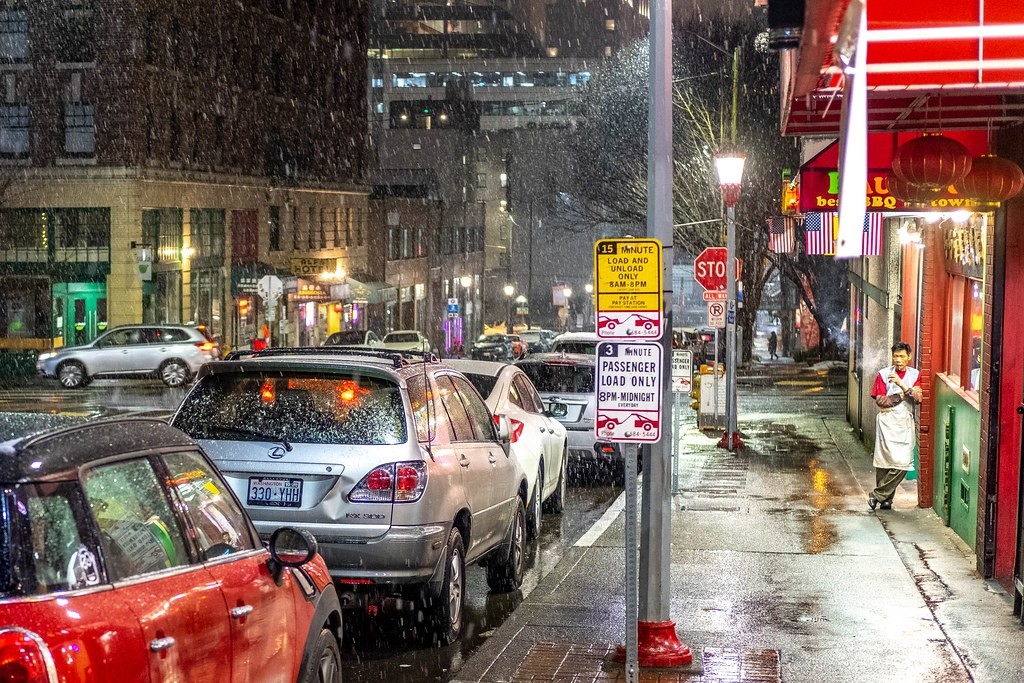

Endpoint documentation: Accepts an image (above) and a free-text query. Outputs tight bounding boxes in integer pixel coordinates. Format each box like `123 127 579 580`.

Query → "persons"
767 331 779 361
867 341 923 511
253 313 271 352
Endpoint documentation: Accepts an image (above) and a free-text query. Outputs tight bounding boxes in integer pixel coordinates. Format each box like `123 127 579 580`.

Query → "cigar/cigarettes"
898 365 900 370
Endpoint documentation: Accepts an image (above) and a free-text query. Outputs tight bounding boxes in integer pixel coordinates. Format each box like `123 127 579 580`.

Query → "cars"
434 356 570 539
700 329 726 362
549 331 644 355
472 334 514 362
1 410 344 683
382 330 431 352
36 323 223 390
320 329 386 348
673 329 708 372
505 335 529 359
168 346 529 645
518 330 556 354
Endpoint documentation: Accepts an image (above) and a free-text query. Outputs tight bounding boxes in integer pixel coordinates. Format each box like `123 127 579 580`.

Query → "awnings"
346 272 397 304
230 259 298 296
290 273 350 302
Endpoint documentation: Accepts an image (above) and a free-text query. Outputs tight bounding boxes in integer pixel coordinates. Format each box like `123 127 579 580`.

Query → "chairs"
275 391 329 429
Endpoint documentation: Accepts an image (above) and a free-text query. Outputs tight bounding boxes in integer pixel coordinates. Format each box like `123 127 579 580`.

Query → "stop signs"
694 247 740 291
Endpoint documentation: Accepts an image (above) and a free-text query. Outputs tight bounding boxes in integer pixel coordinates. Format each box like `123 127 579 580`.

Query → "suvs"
511 349 642 486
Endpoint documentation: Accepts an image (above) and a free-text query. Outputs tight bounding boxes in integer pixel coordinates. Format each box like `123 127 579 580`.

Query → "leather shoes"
869 491 877 510
880 500 891 509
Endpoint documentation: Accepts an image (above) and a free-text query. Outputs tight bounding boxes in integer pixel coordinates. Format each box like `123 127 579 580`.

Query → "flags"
804 210 883 256
766 215 795 254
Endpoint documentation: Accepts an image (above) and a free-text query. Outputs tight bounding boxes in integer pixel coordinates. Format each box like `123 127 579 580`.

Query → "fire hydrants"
689 365 714 428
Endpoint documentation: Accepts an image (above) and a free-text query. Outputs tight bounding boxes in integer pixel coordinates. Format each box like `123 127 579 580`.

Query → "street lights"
715 152 747 450
504 286 514 336
586 284 593 332
564 289 572 332
459 274 472 346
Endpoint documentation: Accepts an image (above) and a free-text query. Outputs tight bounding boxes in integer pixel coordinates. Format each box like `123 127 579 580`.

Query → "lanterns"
892 132 972 188
953 154 1024 210
886 167 948 208
